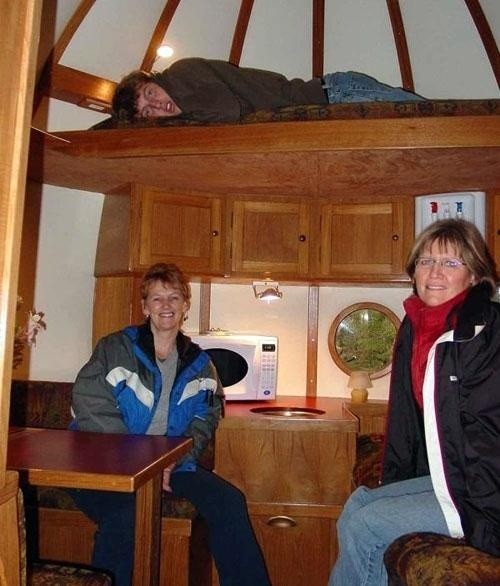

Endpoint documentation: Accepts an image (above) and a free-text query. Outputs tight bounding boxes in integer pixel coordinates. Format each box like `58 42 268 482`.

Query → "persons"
325 220 500 586
67 263 272 586
112 58 427 123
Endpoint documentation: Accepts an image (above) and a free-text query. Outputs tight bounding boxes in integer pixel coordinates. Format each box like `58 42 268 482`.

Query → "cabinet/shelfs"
93 182 500 287
210 429 356 586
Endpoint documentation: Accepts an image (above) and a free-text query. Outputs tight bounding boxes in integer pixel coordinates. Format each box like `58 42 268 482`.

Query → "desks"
6 423 194 586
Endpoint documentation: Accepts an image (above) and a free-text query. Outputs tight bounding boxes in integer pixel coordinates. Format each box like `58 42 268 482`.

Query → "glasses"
414 257 467 270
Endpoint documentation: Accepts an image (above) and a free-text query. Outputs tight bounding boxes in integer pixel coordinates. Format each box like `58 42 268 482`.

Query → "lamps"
253 279 283 304
140 44 173 76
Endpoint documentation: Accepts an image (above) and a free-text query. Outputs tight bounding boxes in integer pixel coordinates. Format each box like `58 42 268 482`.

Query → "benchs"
38 507 191 586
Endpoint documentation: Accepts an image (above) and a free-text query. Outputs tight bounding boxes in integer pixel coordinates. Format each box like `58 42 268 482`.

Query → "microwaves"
182 336 279 401
